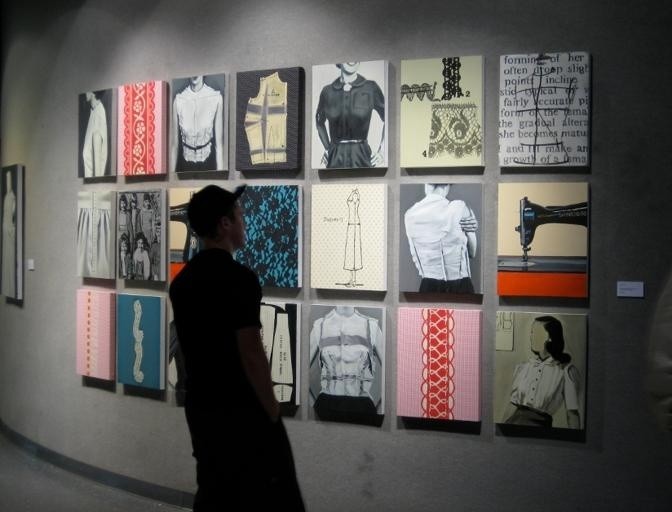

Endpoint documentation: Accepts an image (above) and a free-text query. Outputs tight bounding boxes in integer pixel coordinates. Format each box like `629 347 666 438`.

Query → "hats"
187 185 246 235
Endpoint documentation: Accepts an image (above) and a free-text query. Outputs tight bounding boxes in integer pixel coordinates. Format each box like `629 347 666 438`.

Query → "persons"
308 306 384 414
316 61 385 169
168 183 305 512
501 315 581 431
404 183 478 295
118 193 161 283
82 89 108 178
1 169 16 300
169 75 223 170
